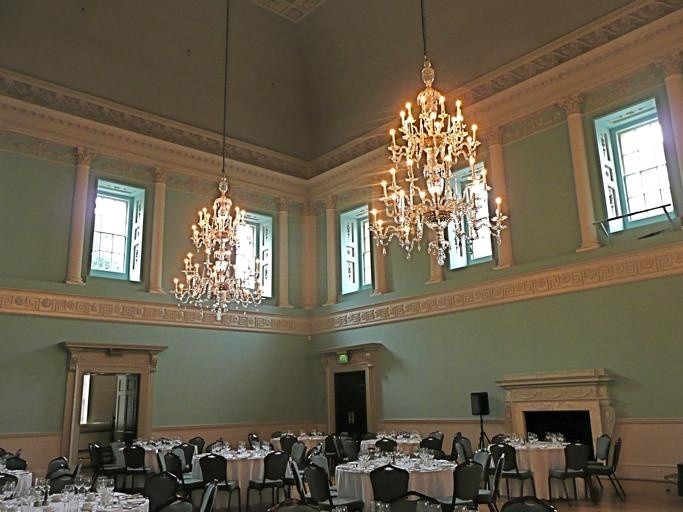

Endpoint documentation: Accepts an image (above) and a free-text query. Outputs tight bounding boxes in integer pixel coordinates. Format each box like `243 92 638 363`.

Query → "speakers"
471 390 492 417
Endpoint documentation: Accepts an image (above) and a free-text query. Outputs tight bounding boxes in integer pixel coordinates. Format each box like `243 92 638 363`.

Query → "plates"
441 464 454 467
423 468 438 470
435 460 446 462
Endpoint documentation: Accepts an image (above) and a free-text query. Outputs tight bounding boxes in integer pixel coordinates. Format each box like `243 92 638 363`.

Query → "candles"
187 252 193 265
405 101 411 116
400 110 405 125
372 208 376 222
381 180 387 196
390 167 396 184
456 98 461 115
195 264 198 272
471 122 477 141
184 259 188 269
439 96 445 112
496 196 502 209
389 128 395 145
198 210 203 221
174 277 179 289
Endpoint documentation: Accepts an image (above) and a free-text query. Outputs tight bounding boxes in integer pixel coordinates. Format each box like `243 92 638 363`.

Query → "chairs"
272 431 283 438
429 449 445 459
207 440 223 452
389 491 439 512
291 461 338 504
0 447 28 470
436 459 483 512
305 463 364 512
285 440 308 497
587 433 611 488
45 456 83 496
375 436 397 452
420 436 442 450
88 440 125 490
189 436 205 454
143 471 179 512
474 448 491 490
429 431 444 441
171 446 193 479
458 436 474 460
370 462 409 504
248 434 262 450
199 453 241 512
343 439 358 461
246 450 289 510
325 433 339 473
478 453 506 512
199 478 218 512
123 444 155 489
153 494 193 512
306 447 318 463
548 440 598 507
446 432 462 461
490 442 536 497
310 456 338 491
500 495 556 512
333 438 349 464
164 452 204 499
456 441 466 463
177 442 195 472
0 472 18 500
267 498 320 512
156 449 165 473
585 437 626 501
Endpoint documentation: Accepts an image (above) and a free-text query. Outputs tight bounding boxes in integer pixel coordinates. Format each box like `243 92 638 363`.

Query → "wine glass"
557 433 561 445
255 442 260 451
430 504 442 512
550 434 557 445
252 441 256 448
366 455 370 461
30 491 43 512
362 449 370 459
60 489 68 512
422 448 429 456
4 481 15 500
413 446 420 459
503 432 507 444
454 505 467 512
74 477 84 494
311 428 316 436
546 432 551 440
530 436 534 448
404 446 410 455
358 451 364 461
365 500 392 512
224 440 232 451
98 476 107 489
512 432 516 440
301 428 306 436
532 434 538 443
428 449 435 459
103 478 115 508
515 433 519 442
262 440 268 449
528 432 532 440
416 500 428 512
336 506 347 512
65 485 75 512
238 440 245 448
507 433 511 441
35 477 43 487
83 475 92 492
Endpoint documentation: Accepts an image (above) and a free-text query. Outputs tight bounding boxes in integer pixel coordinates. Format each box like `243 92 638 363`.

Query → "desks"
117 444 199 489
488 442 590 500
270 436 328 455
0 470 32 495
0 493 149 512
192 452 293 511
361 439 421 454
334 458 458 511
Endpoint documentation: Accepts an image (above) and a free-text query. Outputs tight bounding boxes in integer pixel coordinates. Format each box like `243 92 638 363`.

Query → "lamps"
169 1 263 320
370 0 509 266
